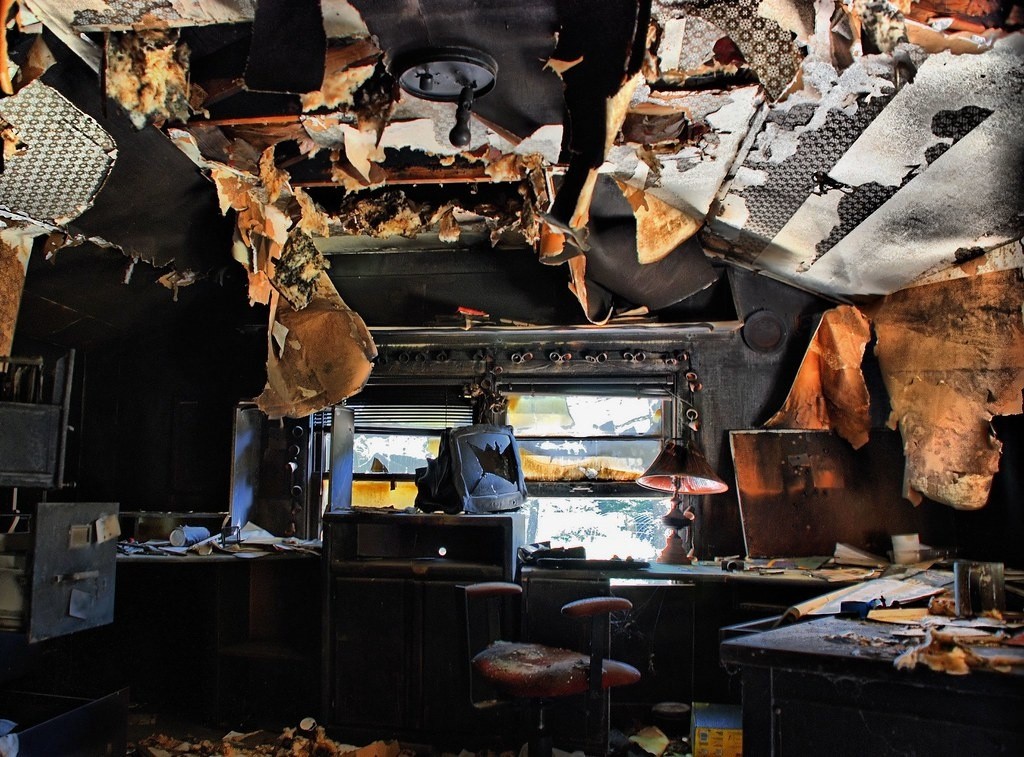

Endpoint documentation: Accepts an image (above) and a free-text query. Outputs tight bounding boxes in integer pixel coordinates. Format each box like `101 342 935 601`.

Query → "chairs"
454 581 641 757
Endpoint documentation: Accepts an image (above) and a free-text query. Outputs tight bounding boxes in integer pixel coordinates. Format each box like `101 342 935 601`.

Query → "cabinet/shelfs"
719 580 1024 757
519 564 731 757
319 576 520 754
